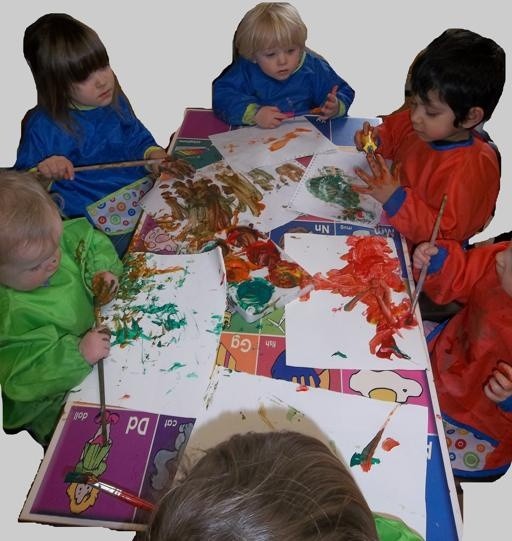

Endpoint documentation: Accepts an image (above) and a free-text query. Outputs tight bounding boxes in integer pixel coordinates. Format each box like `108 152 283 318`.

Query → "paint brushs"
63 472 155 513
405 194 447 324
93 296 106 444
74 157 178 172
276 108 321 120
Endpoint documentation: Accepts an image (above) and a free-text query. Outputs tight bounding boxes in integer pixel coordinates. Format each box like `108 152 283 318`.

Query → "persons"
210 1 355 130
349 27 505 259
0 165 125 447
145 431 377 541
409 238 511 483
13 13 195 258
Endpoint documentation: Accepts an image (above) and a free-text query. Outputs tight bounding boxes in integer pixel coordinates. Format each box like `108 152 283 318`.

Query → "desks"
15 106 467 539
